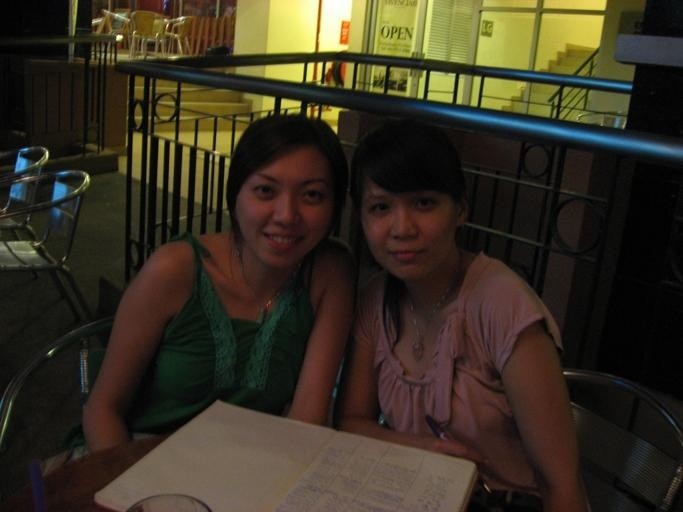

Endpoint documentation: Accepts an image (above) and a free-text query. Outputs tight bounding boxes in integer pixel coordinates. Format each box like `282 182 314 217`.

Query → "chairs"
0 146 49 281
95 8 193 59
378 369 683 511
0 316 111 452
0 169 92 322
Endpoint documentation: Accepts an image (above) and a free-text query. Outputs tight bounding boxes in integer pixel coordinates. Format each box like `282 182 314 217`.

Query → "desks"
1 398 164 512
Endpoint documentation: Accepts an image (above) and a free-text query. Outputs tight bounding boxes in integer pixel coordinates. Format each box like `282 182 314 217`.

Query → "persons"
62 113 361 452
332 113 588 512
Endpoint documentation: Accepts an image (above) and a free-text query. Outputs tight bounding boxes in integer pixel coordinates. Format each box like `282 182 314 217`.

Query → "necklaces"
235 245 304 325
404 248 464 358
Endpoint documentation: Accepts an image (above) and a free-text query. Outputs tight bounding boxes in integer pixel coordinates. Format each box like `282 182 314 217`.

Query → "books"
93 398 480 512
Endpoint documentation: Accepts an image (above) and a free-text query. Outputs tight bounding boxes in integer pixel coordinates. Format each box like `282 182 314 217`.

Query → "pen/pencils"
424 414 494 495
29 459 44 512
